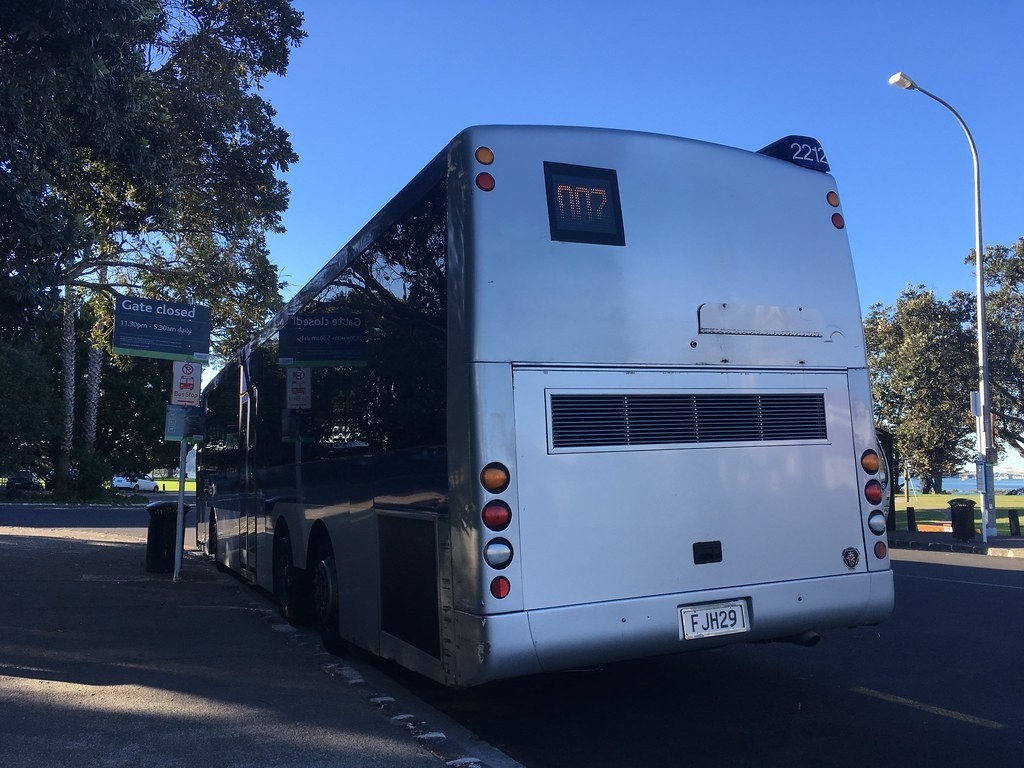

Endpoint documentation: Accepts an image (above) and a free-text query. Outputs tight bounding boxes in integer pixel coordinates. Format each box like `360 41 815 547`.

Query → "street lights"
888 69 997 546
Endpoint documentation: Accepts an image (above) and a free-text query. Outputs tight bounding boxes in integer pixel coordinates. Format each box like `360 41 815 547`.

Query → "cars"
6 469 42 489
45 468 80 491
111 472 159 493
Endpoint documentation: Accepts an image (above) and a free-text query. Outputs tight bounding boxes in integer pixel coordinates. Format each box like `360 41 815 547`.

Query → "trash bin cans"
946 498 978 542
142 499 193 576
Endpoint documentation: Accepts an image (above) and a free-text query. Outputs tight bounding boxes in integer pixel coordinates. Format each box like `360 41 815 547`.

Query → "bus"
193 121 899 696
